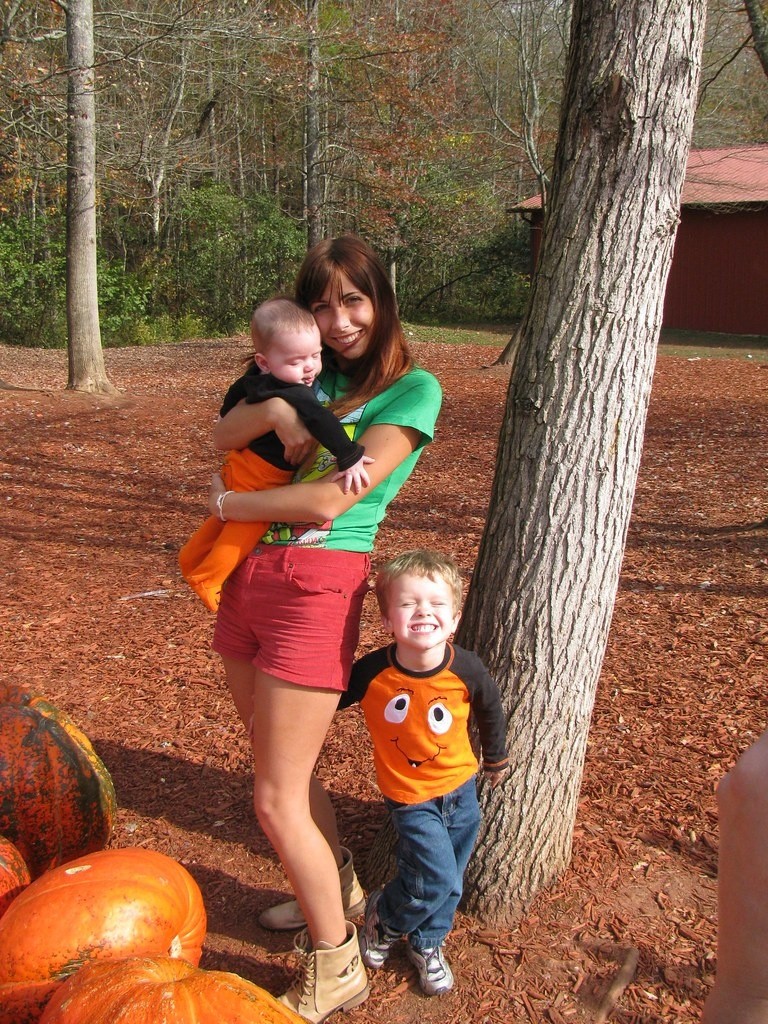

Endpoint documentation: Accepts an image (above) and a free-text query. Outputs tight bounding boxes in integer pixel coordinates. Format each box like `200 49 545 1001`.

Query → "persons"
179 298 376 611
336 549 510 995
209 235 442 1024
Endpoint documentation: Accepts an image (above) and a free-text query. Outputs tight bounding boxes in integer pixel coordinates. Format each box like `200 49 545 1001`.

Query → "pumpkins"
0 679 319 1024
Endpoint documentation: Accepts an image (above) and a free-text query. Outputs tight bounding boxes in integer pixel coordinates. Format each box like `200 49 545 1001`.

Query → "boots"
276 920 370 1024
259 845 366 933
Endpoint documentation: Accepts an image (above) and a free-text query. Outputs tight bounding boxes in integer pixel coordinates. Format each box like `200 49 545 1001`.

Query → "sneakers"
358 889 403 969
406 941 455 996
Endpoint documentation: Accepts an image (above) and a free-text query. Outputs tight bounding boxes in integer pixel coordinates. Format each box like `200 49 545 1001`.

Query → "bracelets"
216 491 235 521
